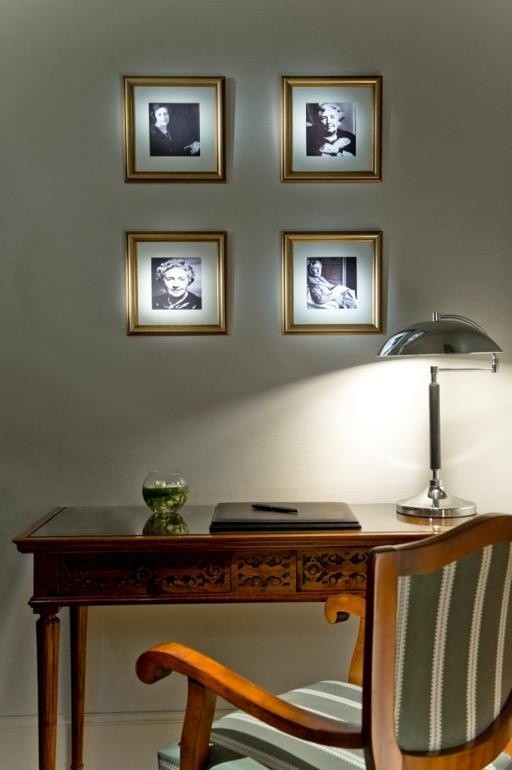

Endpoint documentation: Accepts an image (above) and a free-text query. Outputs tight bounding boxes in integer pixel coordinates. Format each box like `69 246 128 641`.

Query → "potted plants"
142 470 188 512
143 512 188 535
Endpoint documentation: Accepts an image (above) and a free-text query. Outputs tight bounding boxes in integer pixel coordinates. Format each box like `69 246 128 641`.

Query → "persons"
307 103 356 157
152 257 202 310
149 103 200 156
308 260 357 310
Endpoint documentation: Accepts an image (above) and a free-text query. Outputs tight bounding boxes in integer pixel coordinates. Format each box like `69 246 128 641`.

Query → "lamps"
376 313 503 518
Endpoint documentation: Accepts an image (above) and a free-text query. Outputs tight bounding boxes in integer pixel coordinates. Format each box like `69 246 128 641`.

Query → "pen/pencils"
252 504 298 512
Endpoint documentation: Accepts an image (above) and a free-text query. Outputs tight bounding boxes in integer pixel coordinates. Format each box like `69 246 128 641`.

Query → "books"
209 501 362 531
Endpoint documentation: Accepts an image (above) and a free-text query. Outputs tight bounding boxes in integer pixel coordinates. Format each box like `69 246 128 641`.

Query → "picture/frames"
281 75 383 184
281 231 383 334
125 232 227 336
123 77 227 184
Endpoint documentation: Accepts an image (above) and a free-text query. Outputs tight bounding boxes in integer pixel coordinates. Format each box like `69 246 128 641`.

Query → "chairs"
135 512 512 770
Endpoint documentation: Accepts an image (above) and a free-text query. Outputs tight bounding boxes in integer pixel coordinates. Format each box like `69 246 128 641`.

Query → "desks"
12 504 480 770
